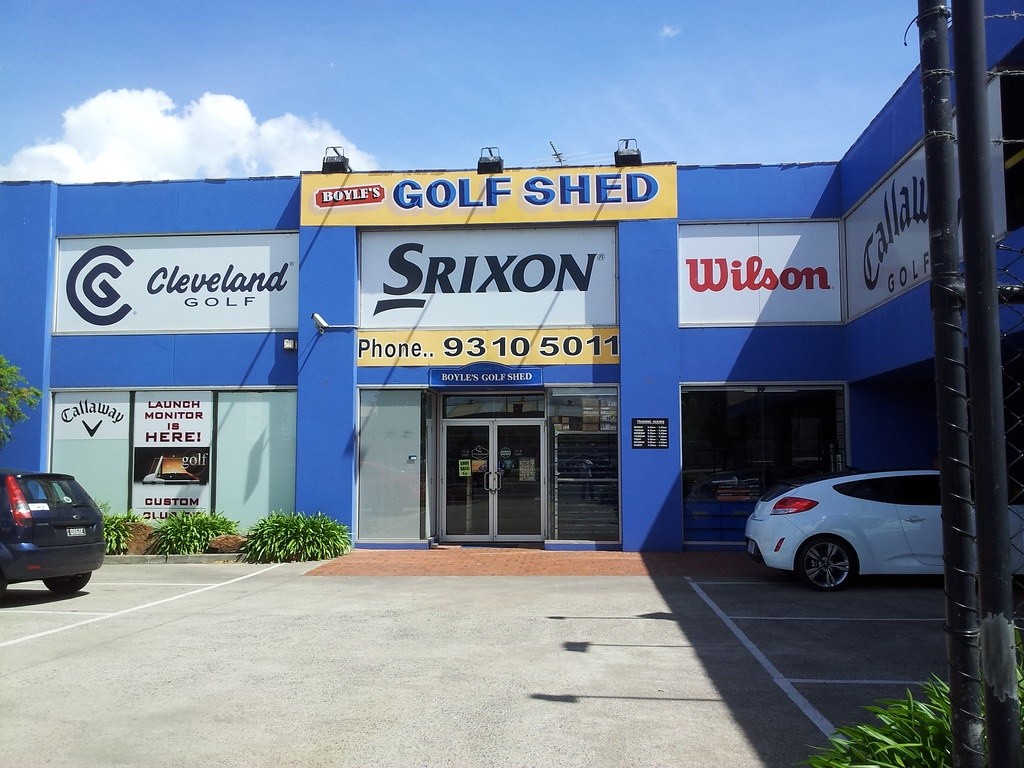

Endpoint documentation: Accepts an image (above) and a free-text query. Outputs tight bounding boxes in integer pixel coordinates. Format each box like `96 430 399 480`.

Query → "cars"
746 471 1024 593
0 468 107 595
685 467 777 502
